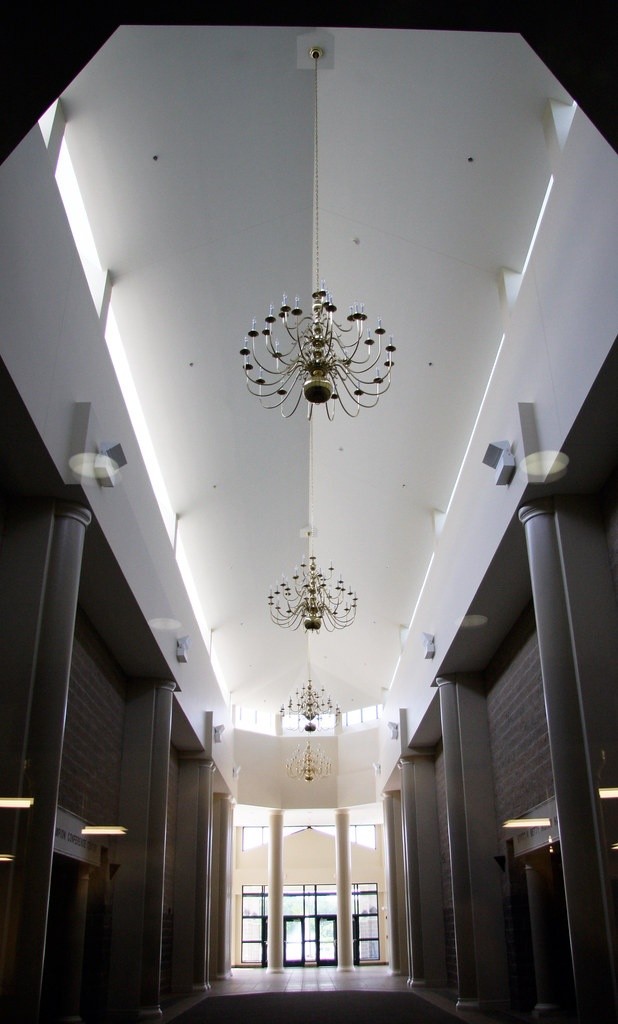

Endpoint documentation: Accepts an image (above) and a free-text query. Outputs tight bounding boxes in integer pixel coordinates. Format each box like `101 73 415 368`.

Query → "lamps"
265 530 357 636
235 31 399 423
285 742 330 783
278 676 344 734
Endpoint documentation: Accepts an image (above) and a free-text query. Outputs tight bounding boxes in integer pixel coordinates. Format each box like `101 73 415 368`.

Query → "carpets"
171 990 472 1024
